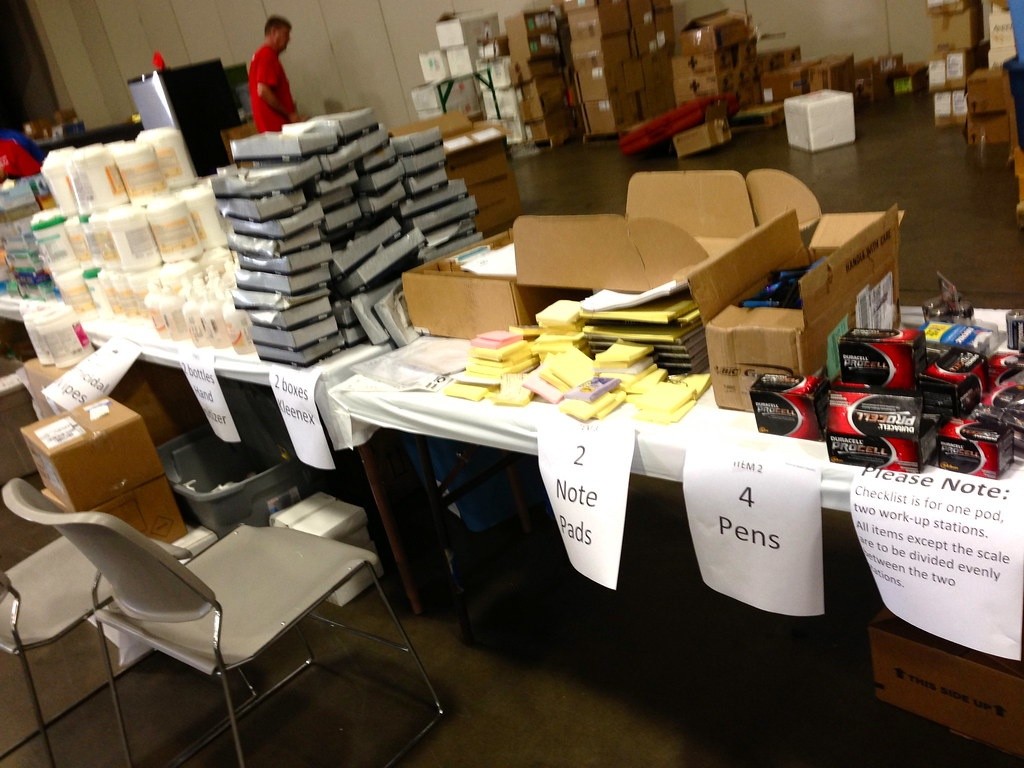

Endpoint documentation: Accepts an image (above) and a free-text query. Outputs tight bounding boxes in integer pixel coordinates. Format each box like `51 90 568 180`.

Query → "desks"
1 293 423 617
339 325 1024 643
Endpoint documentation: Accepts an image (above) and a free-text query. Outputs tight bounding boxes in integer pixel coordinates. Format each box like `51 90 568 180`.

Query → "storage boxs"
0 0 1024 768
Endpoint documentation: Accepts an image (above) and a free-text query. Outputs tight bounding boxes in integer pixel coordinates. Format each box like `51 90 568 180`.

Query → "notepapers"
442 299 713 426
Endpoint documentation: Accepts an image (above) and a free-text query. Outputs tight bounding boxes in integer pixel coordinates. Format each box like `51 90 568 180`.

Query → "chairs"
0 533 256 768
0 477 446 767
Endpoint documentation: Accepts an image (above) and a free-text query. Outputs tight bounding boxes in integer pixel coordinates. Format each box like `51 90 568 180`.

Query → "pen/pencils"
739 299 781 310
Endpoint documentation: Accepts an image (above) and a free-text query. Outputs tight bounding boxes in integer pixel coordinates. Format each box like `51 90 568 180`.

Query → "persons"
247 15 307 134
0 129 45 183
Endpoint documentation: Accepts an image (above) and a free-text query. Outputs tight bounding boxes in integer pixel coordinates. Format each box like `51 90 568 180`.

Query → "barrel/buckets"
22 130 258 369
